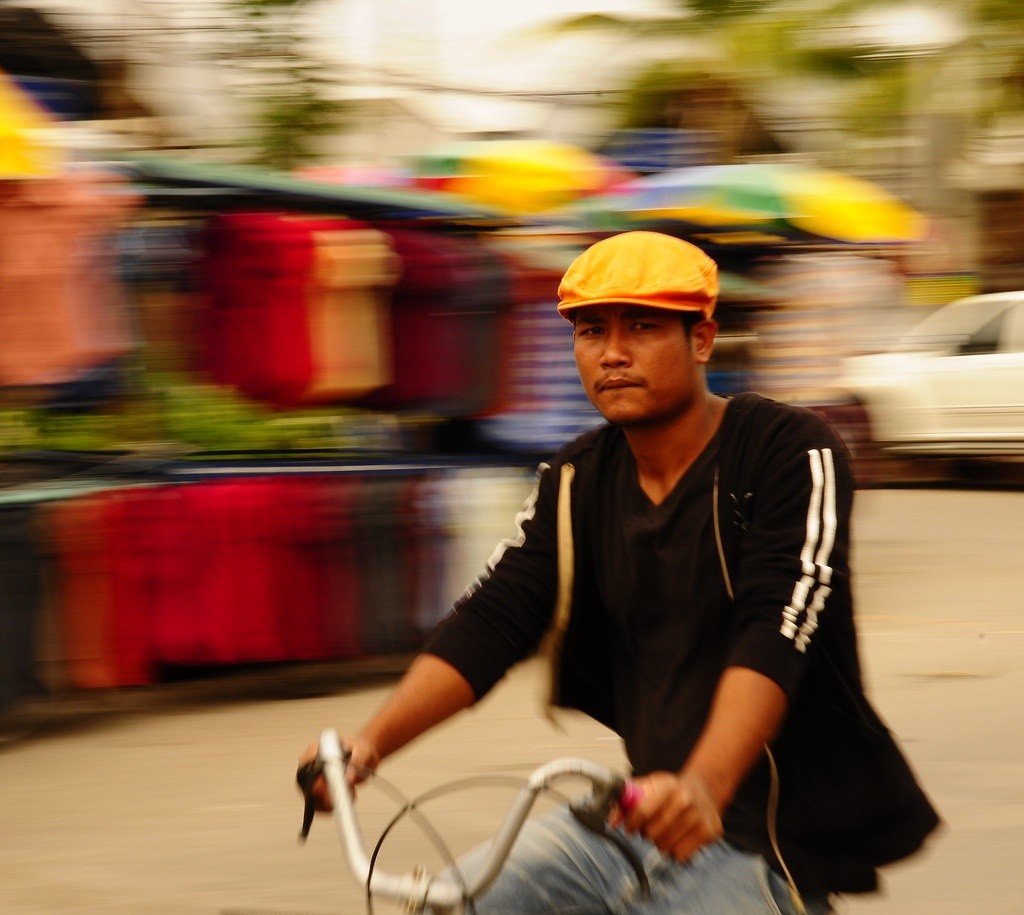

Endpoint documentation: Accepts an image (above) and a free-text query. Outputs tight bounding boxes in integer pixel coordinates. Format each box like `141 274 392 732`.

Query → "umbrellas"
387 136 934 248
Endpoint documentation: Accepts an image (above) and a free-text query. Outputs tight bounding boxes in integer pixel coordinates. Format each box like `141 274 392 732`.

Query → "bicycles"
296 730 695 915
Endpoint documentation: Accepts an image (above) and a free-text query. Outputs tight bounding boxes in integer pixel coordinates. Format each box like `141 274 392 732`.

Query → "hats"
556 230 719 326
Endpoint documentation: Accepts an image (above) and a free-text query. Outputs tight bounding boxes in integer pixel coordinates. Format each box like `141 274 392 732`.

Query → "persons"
295 230 941 915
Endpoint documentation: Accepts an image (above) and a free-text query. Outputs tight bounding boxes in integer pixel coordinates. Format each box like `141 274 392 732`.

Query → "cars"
814 291 1024 488
